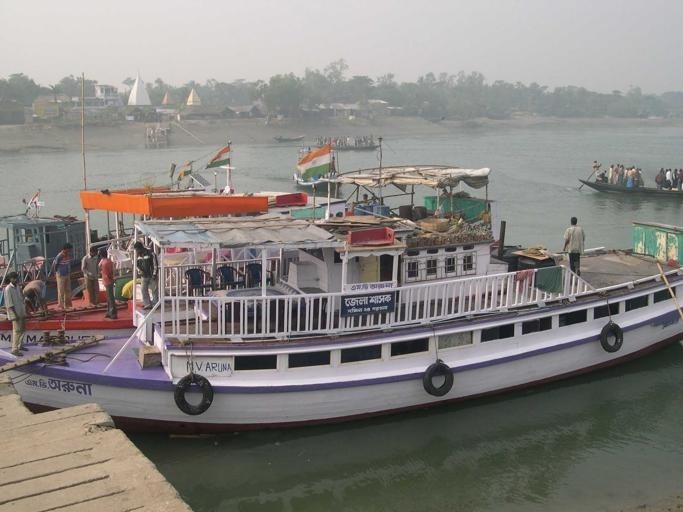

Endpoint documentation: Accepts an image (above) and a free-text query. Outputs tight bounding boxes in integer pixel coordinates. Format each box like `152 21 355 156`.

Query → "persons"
81 246 117 319
361 194 371 206
655 167 683 191
23 280 46 316
134 242 158 309
330 157 335 177
563 217 585 286
4 272 29 356
54 244 72 310
316 135 367 147
592 161 644 188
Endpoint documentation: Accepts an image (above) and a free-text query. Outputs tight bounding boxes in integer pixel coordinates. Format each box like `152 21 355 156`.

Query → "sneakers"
104 313 118 320
11 347 28 357
142 304 156 310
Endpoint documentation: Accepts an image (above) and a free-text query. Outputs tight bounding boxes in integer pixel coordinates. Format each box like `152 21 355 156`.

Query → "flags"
208 145 230 168
178 162 192 178
297 145 331 179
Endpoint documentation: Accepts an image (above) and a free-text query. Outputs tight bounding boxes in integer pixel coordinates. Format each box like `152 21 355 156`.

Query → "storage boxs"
399 206 426 219
493 246 556 271
85 289 106 303
354 206 390 216
